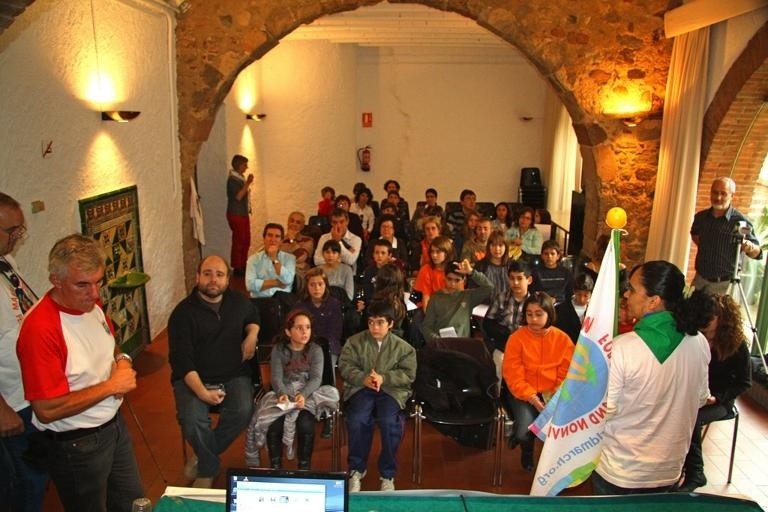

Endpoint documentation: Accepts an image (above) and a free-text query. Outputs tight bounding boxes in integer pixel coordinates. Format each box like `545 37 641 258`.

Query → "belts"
701 275 733 283
46 413 117 440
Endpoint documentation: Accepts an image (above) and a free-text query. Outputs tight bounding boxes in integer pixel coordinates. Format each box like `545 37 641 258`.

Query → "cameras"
730 215 748 242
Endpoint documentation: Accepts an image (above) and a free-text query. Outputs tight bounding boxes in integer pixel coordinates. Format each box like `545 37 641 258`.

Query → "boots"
297 433 315 470
266 426 283 471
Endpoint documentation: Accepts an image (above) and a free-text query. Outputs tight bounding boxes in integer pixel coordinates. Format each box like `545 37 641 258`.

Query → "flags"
527 230 621 499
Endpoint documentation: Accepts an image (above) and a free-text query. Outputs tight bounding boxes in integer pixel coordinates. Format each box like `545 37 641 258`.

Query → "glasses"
10 273 24 299
4 225 28 240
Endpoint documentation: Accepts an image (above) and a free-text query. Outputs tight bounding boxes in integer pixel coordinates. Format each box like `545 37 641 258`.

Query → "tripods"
717 240 768 379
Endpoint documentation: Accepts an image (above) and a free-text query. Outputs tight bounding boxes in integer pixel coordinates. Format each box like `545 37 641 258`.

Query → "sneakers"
348 470 362 491
508 435 519 450
379 476 395 492
520 451 534 471
192 477 213 489
678 468 707 491
320 419 334 439
183 453 199 480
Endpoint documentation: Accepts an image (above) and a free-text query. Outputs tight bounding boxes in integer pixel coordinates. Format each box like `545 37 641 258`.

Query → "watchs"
749 241 756 254
272 259 282 265
115 350 136 365
287 238 297 244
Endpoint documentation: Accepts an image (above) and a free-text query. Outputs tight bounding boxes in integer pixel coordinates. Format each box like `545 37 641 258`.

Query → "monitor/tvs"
225 468 349 512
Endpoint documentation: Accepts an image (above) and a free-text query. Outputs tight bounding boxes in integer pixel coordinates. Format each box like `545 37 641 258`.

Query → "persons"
226 154 254 278
293 267 344 440
503 208 544 262
410 188 444 225
264 308 324 478
454 210 481 260
368 214 408 261
0 193 52 512
690 178 763 294
343 239 407 338
318 187 335 220
353 182 380 219
503 290 577 472
592 263 712 497
461 217 493 265
302 238 354 303
380 178 409 216
484 265 531 355
617 269 638 335
465 231 511 289
245 223 296 318
359 262 408 330
333 195 363 238
16 231 144 512
420 215 442 268
421 260 495 344
413 236 459 315
490 202 513 232
387 191 405 219
534 207 556 240
167 256 262 488
280 212 315 271
314 209 363 283
672 292 753 493
445 189 483 235
380 203 404 230
338 301 417 494
348 188 375 240
531 239 573 306
555 274 594 345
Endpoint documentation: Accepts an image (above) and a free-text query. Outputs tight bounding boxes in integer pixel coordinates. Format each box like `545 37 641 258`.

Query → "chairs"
310 334 338 474
178 296 282 469
698 404 740 484
409 336 508 488
